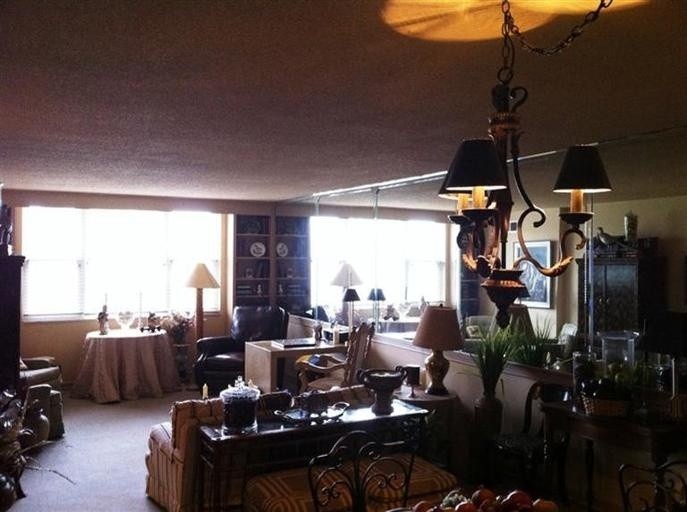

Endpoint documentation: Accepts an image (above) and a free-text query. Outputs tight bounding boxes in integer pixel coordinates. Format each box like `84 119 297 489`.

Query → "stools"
47 389 65 439
341 452 459 511
244 464 355 511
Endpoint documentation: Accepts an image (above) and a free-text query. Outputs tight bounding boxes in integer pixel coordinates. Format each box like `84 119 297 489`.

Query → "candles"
202 382 208 398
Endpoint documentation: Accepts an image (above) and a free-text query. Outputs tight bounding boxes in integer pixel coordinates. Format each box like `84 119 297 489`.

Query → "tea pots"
293 389 328 417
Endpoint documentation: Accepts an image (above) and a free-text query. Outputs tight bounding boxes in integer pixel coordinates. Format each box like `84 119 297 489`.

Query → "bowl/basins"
115 315 135 333
638 237 658 255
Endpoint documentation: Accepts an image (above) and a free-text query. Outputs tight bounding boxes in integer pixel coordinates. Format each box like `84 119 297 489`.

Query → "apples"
580 378 611 398
411 488 560 512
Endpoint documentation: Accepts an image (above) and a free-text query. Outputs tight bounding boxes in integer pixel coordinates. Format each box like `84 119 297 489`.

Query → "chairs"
304 430 414 511
461 315 493 352
18 350 64 408
492 378 574 510
614 458 686 512
538 321 577 359
191 302 289 398
294 320 375 394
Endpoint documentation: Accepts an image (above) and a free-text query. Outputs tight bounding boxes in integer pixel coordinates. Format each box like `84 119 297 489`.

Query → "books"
272 338 316 348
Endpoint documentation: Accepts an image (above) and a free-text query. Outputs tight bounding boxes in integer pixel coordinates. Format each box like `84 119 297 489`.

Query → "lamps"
183 263 223 340
409 303 464 396
486 304 538 346
511 146 613 276
342 287 361 332
368 289 391 332
437 1 619 310
330 265 363 313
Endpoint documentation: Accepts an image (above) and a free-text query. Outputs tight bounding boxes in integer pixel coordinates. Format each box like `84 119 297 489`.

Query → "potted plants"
464 313 524 445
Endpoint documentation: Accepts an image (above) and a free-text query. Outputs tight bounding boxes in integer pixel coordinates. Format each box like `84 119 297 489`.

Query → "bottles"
220 374 260 430
256 284 263 295
277 284 283 295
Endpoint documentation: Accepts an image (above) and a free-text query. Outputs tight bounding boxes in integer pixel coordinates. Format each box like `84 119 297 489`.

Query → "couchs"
144 384 377 511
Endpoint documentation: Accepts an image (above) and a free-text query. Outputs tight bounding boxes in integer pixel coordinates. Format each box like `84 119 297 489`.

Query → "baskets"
581 392 632 420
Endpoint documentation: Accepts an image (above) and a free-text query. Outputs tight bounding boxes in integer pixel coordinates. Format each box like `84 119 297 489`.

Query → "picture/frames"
511 239 553 311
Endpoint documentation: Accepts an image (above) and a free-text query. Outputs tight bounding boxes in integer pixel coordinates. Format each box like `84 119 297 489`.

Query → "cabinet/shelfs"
225 213 270 330
570 258 668 351
271 215 312 319
0 255 25 394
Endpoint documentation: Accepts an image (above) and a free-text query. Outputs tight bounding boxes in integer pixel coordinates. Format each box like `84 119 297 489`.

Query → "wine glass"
355 366 409 415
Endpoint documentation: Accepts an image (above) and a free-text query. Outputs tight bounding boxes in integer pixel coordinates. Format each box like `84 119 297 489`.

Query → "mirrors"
270 123 686 395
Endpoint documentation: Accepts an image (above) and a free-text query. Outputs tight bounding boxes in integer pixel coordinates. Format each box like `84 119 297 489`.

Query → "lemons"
607 362 637 383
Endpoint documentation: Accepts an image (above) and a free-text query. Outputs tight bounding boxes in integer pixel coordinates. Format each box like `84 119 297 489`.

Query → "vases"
174 342 191 383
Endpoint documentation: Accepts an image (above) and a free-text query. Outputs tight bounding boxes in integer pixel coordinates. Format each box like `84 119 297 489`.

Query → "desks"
189 395 429 510
390 381 459 470
535 400 686 511
373 316 421 334
85 327 168 403
242 337 348 394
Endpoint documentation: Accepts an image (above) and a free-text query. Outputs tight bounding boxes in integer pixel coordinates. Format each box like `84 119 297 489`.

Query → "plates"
248 241 266 257
245 219 261 234
275 241 288 257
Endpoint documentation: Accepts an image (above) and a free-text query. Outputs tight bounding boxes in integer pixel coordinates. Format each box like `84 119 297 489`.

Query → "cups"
597 330 641 379
623 215 637 240
571 351 596 412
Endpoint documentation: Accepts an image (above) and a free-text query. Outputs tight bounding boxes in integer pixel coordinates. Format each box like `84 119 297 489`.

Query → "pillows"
19 358 26 369
168 396 223 454
480 322 490 338
468 323 482 338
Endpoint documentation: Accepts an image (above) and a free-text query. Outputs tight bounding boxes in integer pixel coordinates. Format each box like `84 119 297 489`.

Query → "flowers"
162 311 207 343
